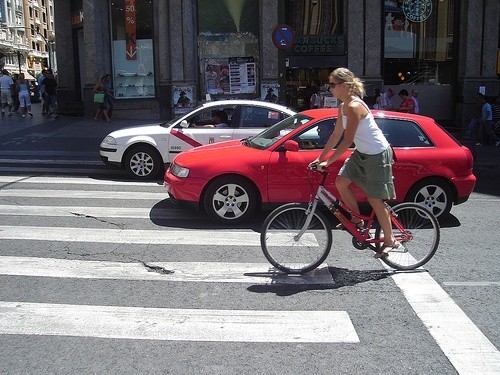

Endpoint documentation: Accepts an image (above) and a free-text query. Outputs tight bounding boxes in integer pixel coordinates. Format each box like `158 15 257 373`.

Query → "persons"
365 85 420 115
0 68 34 118
300 85 321 110
177 91 191 104
307 67 406 259
463 92 500 147
206 66 217 89
38 69 59 118
91 74 114 121
302 121 336 149
192 110 229 129
220 65 229 87
264 88 278 101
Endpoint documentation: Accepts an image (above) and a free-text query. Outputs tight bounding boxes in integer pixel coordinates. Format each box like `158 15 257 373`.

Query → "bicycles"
260 163 441 275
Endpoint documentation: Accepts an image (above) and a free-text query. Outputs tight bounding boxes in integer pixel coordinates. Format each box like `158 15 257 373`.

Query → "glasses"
330 82 343 89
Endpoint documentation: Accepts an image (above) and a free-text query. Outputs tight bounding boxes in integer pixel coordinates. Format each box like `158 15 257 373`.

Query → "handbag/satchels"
94 92 105 103
389 143 399 165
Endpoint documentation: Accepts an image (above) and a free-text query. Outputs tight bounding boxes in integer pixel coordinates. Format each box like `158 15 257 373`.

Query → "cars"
99 99 320 181
162 107 476 227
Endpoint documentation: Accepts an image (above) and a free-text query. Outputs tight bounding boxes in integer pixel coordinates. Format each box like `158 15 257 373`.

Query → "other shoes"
28 111 33 116
22 114 25 118
1 109 5 114
8 112 12 116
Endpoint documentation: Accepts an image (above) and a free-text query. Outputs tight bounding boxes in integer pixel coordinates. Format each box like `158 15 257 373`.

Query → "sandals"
336 220 364 230
374 240 400 258
